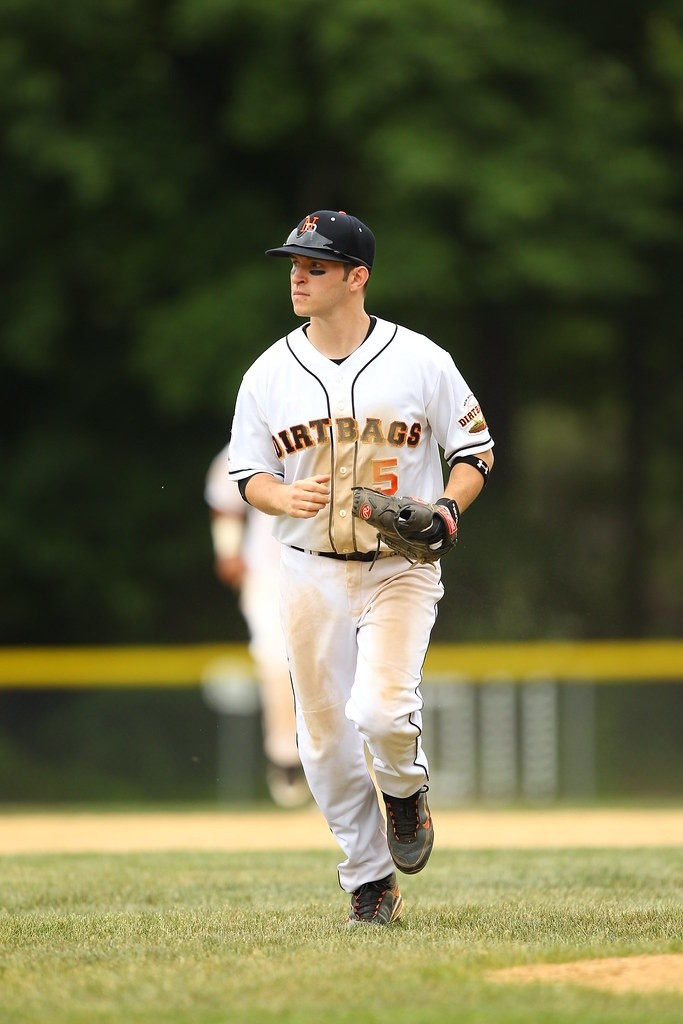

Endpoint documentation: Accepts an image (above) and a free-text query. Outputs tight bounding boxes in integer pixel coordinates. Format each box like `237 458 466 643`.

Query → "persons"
228 211 495 928
204 445 308 808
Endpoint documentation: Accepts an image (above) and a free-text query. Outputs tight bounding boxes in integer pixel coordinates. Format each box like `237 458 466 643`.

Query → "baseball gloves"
349 485 458 572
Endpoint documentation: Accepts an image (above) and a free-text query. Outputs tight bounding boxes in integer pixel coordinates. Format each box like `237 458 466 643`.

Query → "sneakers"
347 870 405 933
383 785 436 881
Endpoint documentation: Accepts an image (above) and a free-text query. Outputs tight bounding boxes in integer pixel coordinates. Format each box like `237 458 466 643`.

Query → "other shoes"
265 764 309 807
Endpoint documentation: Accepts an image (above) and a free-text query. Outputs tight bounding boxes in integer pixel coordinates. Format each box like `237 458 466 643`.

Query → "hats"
263 210 377 276
